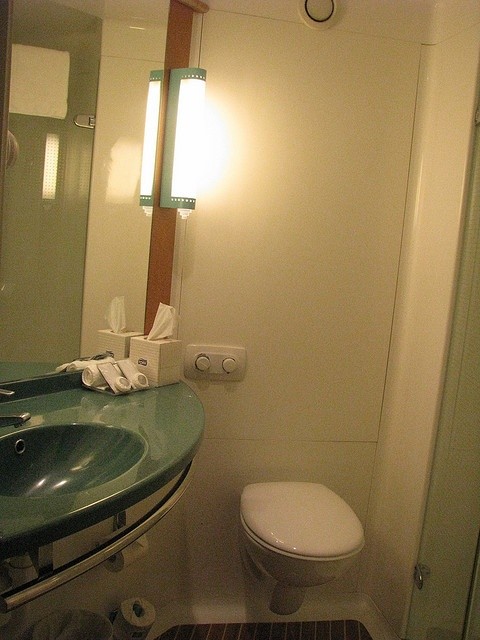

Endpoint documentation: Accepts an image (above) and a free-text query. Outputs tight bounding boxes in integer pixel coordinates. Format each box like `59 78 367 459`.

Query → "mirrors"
1 0 103 385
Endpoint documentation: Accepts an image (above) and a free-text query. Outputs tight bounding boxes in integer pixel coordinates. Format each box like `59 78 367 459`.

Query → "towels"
82 364 122 386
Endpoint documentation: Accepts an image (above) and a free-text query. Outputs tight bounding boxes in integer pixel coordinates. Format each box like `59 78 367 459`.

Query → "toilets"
239 480 365 616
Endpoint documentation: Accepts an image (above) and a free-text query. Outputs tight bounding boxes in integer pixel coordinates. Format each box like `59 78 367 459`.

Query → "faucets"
0 385 32 429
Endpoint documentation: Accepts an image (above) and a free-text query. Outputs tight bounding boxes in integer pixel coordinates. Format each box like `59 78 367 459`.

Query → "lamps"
140 67 161 208
159 68 207 211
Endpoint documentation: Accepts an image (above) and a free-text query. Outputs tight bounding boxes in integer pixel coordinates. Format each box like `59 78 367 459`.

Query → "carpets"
155 619 368 640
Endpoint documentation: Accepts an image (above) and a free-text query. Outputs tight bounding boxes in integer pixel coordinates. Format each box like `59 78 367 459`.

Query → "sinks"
0 373 206 558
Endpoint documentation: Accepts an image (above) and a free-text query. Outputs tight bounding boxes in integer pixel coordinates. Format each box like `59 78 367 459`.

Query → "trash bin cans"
32 608 114 640
